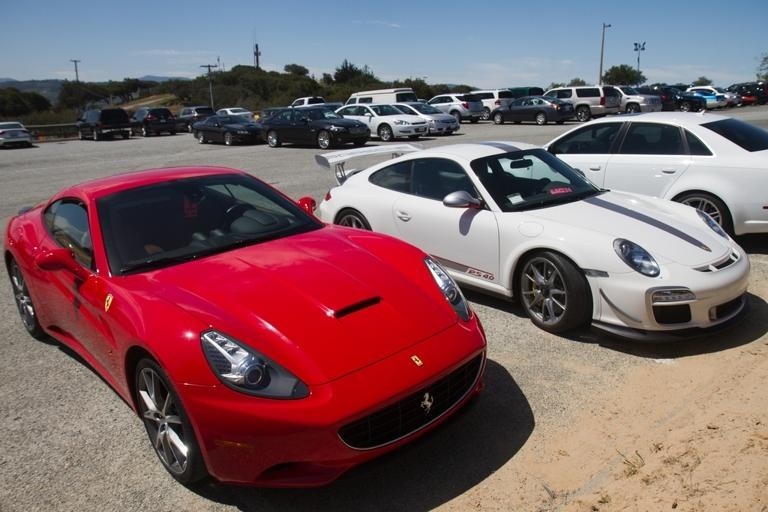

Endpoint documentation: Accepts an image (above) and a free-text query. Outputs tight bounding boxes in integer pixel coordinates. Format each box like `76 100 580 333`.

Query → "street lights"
199 64 218 110
69 59 81 82
598 21 612 85
632 41 647 87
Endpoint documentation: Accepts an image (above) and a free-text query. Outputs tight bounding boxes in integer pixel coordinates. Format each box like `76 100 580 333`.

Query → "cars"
406 101 461 137
646 82 767 112
261 105 373 150
425 92 484 124
485 109 767 237
417 99 427 103
214 107 255 121
333 102 431 142
0 121 33 149
286 96 327 108
189 114 263 148
487 94 577 127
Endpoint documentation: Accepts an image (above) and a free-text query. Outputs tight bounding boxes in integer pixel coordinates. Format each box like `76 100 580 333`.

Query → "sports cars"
3 163 491 493
313 138 750 345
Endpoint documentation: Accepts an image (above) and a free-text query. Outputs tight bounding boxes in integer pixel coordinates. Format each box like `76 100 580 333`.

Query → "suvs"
173 106 217 134
129 106 178 137
75 107 132 142
611 83 663 114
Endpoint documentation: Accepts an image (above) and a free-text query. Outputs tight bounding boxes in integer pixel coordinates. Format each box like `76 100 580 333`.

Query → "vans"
343 87 418 106
540 85 621 124
467 88 516 121
